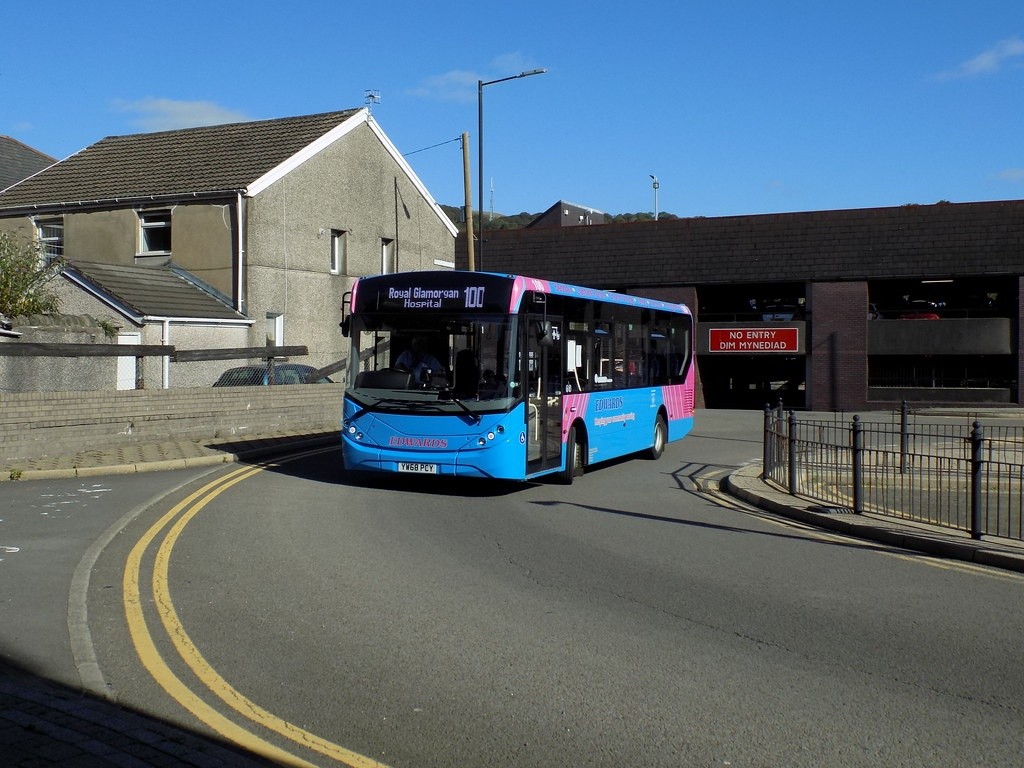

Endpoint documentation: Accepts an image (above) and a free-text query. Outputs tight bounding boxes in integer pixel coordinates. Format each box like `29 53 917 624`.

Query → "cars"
868 304 883 321
896 300 942 319
762 299 800 321
212 364 334 387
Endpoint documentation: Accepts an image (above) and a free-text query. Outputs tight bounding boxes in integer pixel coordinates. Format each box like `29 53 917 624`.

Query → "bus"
338 271 696 486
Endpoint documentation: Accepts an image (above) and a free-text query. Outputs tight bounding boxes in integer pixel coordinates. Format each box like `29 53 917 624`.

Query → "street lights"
479 69 547 271
650 175 659 220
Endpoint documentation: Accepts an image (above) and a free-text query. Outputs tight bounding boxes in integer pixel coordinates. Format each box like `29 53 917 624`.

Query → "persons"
396 339 446 385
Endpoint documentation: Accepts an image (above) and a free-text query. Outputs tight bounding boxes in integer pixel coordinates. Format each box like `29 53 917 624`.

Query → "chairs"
455 349 480 396
